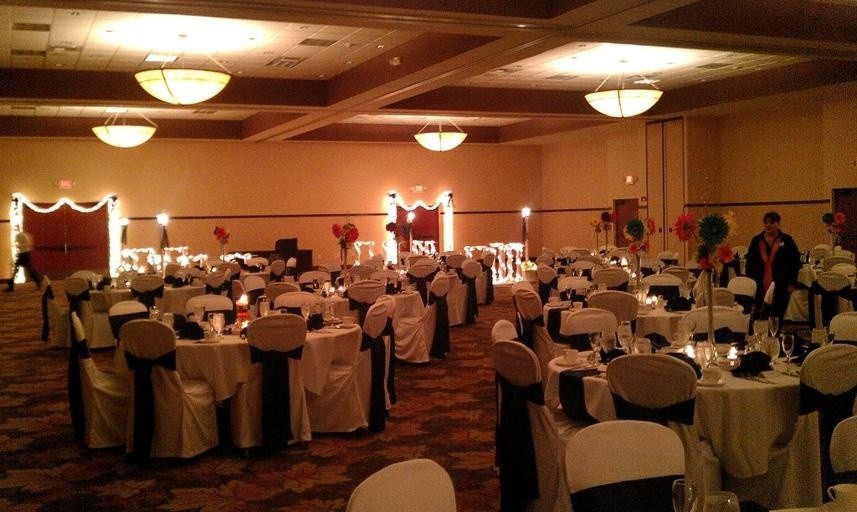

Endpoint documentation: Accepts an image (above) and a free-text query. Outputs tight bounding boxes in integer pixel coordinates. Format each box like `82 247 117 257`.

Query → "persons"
746 213 800 336
3 225 42 292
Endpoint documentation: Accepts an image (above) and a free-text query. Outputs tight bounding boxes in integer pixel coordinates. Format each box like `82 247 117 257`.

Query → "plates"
548 303 563 307
698 379 726 387
556 359 581 367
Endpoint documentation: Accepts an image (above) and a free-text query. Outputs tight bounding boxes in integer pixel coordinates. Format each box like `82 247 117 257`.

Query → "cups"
548 297 559 305
702 367 722 383
561 347 579 363
827 483 855 499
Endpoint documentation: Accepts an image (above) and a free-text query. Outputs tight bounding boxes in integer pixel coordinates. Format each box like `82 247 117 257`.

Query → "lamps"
92 107 157 148
583 66 661 117
133 46 231 105
414 115 467 153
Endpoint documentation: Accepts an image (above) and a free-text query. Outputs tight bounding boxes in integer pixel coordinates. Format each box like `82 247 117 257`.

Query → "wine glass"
748 317 796 376
588 323 652 356
149 298 336 339
672 478 706 512
565 287 573 303
704 490 741 511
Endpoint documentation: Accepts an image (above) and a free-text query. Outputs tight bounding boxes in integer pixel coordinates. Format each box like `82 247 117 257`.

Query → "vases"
605 231 607 256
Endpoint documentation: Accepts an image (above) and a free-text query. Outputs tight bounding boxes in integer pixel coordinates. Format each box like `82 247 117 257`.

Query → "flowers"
213 226 229 244
331 222 359 250
386 221 410 242
822 212 847 234
590 211 618 234
623 217 655 254
673 211 738 274
